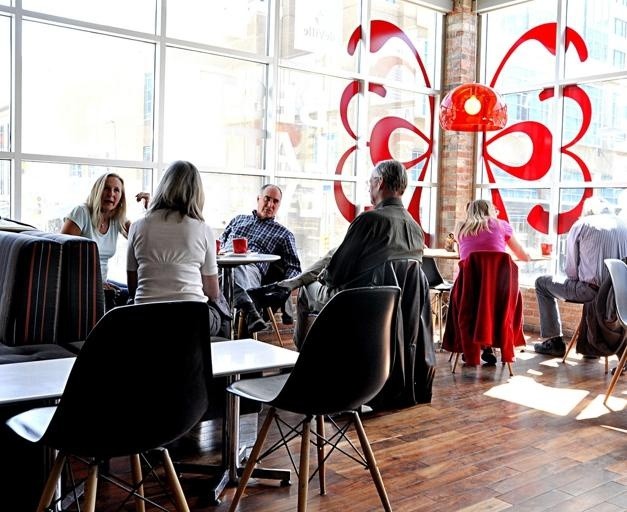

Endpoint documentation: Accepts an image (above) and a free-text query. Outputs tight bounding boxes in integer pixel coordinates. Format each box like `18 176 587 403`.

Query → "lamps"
439 12 507 131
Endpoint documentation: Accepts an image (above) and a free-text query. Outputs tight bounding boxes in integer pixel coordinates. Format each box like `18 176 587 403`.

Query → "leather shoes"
246 281 290 305
248 310 267 333
481 347 496 363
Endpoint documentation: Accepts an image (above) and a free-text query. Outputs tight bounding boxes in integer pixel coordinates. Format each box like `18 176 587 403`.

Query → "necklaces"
100 223 110 230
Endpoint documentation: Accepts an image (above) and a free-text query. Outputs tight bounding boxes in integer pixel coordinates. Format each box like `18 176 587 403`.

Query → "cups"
541 243 552 255
233 238 248 254
216 240 220 252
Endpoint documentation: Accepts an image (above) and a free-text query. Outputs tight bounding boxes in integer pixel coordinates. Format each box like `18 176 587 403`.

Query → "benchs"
0 214 109 354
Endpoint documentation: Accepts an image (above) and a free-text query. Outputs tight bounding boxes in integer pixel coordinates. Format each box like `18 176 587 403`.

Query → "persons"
440 200 531 366
127 161 233 341
61 172 153 313
534 195 627 356
217 184 302 380
246 160 425 375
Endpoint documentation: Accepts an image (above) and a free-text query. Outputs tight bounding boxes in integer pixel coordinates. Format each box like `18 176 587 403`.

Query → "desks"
1 334 300 505
213 253 282 340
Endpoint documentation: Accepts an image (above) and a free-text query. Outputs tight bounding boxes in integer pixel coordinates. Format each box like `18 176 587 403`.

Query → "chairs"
439 251 520 377
223 284 402 512
559 273 611 376
6 300 207 511
421 256 453 350
234 261 287 347
604 259 626 406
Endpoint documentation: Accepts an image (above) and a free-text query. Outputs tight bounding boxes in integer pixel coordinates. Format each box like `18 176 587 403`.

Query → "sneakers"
535 339 565 356
